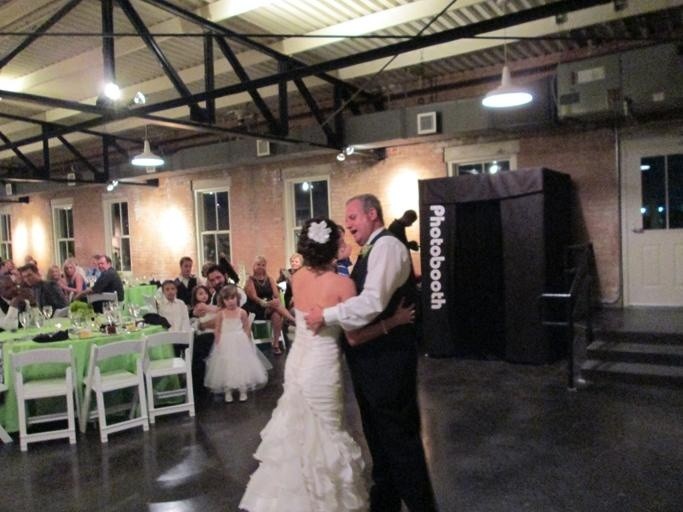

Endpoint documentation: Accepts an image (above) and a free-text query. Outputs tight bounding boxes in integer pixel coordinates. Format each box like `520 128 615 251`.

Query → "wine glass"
68 302 145 339
20 306 52 330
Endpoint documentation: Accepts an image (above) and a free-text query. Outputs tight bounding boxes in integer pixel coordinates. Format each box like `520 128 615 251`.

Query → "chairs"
9 344 79 452
250 319 287 350
0 384 14 444
142 328 196 424
80 335 150 443
86 291 118 305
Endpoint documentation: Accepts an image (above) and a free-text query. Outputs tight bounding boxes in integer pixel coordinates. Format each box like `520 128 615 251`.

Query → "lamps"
96 82 121 106
336 144 354 161
126 92 146 107
131 123 165 167
482 0 533 109
106 177 119 192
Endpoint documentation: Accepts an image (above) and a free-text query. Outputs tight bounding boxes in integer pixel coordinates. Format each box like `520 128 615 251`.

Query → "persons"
303 191 440 512
199 400 276 488
388 210 420 253
234 214 418 511
0 223 354 402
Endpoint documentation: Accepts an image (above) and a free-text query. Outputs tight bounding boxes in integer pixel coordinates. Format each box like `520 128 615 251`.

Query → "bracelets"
380 317 389 335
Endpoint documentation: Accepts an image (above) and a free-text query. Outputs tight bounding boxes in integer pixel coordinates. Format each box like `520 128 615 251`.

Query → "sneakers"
271 346 283 355
239 394 250 402
225 392 234 402
